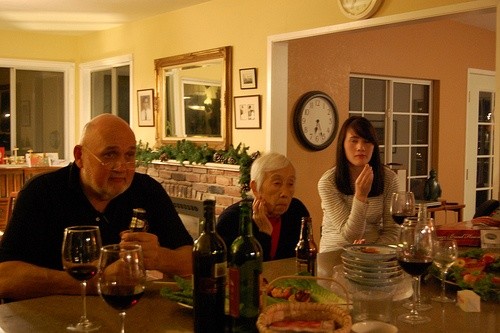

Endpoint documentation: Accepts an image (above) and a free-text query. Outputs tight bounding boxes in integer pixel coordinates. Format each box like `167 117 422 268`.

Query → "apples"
454 257 466 267
463 274 477 282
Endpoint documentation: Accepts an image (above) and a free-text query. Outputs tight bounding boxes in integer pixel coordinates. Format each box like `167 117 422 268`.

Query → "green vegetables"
160 275 193 305
263 271 353 310
446 250 500 302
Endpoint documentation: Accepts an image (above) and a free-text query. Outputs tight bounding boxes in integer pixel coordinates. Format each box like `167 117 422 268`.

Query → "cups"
390 191 414 226
351 321 398 333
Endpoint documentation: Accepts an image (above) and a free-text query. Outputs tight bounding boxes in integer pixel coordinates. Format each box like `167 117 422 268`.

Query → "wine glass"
395 224 433 326
432 238 458 303
403 217 432 312
97 244 145 333
61 225 105 332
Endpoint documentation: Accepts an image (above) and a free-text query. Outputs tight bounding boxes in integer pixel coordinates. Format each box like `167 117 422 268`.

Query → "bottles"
192 200 227 333
130 208 147 232
417 203 427 224
295 217 318 277
227 202 263 333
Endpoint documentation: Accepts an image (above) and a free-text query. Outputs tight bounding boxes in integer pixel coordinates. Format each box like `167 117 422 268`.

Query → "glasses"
84 147 138 172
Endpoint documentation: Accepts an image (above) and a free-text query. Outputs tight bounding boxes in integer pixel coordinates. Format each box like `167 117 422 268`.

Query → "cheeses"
457 290 481 312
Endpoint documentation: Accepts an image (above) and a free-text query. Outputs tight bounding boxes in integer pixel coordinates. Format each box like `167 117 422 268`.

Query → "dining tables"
0 246 500 333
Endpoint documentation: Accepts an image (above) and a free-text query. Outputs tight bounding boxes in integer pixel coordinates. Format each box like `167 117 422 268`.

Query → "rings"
253 212 255 213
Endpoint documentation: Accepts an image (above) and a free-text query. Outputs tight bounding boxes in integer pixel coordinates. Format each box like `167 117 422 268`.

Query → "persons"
141 97 150 120
317 116 403 254
0 113 194 303
215 153 314 267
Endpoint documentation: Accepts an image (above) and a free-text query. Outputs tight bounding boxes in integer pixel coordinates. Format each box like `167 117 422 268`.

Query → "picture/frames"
239 68 257 89
137 89 155 128
21 100 32 128
369 120 397 154
234 95 261 130
154 45 232 151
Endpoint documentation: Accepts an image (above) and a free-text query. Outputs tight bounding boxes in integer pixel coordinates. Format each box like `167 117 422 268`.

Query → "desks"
428 204 466 222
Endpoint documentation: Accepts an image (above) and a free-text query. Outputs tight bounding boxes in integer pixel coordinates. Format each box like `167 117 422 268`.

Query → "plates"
336 242 406 292
177 301 194 308
433 273 463 288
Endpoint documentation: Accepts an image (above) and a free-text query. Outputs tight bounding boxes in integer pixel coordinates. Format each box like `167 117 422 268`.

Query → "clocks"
338 0 382 20
293 91 340 152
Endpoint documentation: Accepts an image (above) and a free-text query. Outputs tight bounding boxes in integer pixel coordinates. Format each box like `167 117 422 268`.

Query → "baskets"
256 274 352 333
